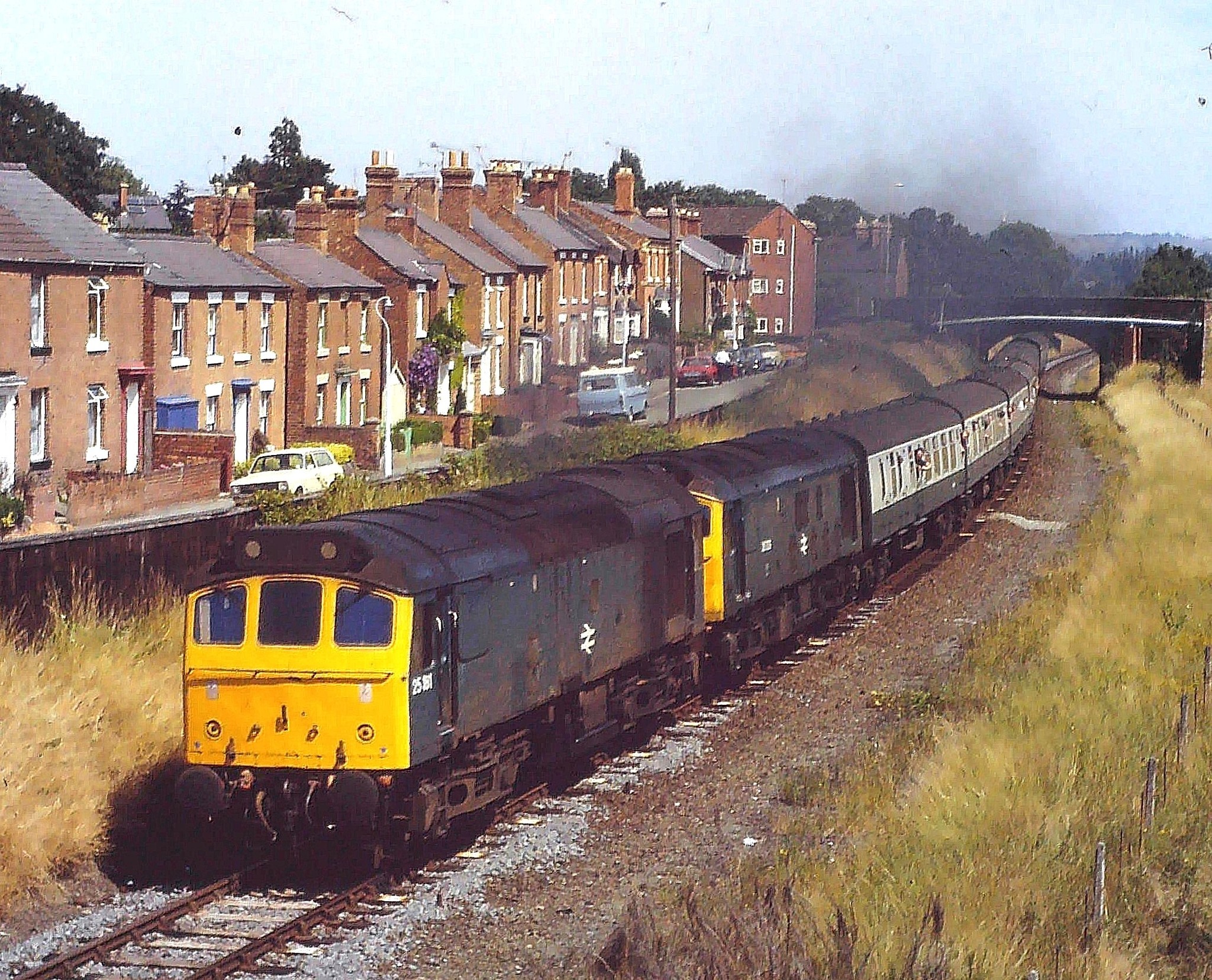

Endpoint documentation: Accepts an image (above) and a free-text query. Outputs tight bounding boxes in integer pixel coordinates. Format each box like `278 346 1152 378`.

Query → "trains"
173 333 1042 876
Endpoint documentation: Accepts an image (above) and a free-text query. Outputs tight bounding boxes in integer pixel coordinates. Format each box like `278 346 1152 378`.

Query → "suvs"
575 366 651 424
229 447 347 506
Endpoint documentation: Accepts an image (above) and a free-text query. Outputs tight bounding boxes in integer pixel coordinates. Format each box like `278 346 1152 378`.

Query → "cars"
676 341 795 388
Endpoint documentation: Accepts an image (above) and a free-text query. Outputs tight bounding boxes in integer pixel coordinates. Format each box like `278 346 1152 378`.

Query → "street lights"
375 295 395 480
885 183 905 274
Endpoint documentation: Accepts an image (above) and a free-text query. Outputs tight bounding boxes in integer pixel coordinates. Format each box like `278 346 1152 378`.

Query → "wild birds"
705 21 712 33
1194 94 1208 108
330 4 358 23
660 1 668 7
1199 42 1212 62
